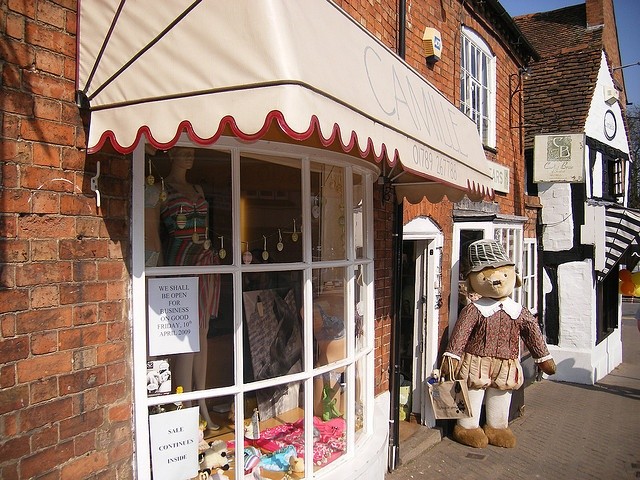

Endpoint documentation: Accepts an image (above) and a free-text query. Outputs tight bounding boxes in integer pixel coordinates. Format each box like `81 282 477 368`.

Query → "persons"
145 145 222 432
244 407 261 448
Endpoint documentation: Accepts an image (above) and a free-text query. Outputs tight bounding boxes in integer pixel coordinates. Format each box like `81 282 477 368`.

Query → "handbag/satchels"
427 356 473 419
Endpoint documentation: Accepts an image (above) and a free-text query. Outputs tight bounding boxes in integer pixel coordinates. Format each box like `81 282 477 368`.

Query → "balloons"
619 269 640 297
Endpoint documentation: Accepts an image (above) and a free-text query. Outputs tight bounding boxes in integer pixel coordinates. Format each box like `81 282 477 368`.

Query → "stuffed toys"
244 444 305 480
198 440 230 475
426 368 445 387
441 238 558 449
